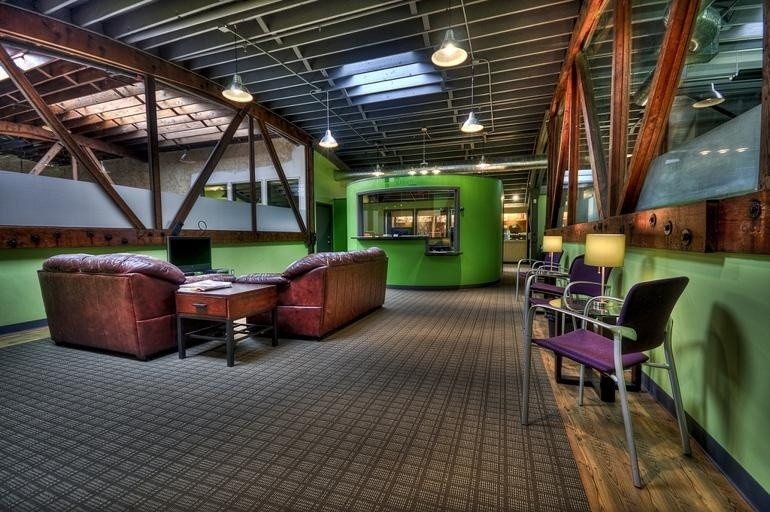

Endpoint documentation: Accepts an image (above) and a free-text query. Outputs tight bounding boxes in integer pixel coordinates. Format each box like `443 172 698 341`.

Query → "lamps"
370 129 443 176
461 78 484 134
692 81 726 109
477 155 490 170
543 234 562 273
318 91 338 149
585 234 625 307
431 0 469 69
664 0 721 56
220 29 254 103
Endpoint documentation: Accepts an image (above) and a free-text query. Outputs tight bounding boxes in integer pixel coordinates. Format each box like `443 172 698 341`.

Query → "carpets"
1 267 597 511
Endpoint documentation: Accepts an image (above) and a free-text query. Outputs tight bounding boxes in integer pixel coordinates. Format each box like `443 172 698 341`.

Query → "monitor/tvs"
167 234 212 275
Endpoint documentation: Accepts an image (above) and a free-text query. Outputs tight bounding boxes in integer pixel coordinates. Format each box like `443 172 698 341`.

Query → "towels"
177 279 232 292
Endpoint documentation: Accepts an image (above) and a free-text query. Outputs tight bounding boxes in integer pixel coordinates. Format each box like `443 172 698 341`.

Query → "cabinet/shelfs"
175 282 279 368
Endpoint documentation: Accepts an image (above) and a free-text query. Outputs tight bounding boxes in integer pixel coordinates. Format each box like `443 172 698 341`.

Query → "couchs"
37 254 236 363
236 245 388 341
